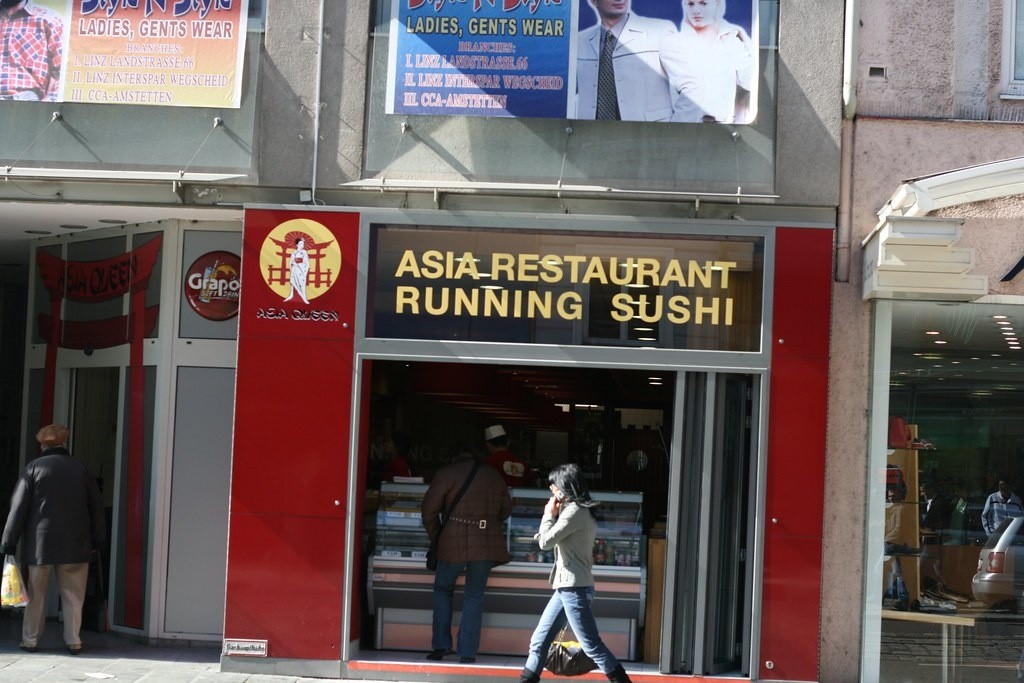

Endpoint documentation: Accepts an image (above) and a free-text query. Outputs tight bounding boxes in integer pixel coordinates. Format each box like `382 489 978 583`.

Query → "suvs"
971 516 1024 611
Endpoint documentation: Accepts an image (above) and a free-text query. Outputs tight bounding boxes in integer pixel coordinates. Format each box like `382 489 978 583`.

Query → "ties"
597 30 617 120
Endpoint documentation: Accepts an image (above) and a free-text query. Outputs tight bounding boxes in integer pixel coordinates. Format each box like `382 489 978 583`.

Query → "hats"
36 424 69 446
485 425 506 441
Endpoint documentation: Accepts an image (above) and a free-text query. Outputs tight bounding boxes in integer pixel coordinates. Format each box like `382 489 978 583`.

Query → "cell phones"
554 491 565 504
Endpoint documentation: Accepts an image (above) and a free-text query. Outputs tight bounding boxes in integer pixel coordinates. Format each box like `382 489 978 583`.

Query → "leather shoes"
911 436 935 450
918 590 958 612
69 644 82 654
885 541 923 555
19 641 37 652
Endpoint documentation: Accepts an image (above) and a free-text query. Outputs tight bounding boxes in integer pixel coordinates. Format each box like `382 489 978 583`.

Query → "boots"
605 662 633 683
518 666 540 683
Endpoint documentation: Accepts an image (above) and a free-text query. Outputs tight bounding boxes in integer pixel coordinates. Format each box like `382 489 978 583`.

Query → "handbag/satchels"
425 538 438 571
883 557 909 611
543 622 598 677
886 464 908 502
1 555 29 608
888 416 912 449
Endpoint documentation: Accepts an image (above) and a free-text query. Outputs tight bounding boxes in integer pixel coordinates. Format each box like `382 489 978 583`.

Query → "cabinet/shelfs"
882 423 998 683
366 479 648 664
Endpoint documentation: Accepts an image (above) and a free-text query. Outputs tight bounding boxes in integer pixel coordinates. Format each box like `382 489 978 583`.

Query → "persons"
919 476 1024 602
520 463 631 683
380 430 413 482
420 432 513 664
884 488 908 612
0 0 65 102
0 424 107 655
483 425 529 486
576 0 750 124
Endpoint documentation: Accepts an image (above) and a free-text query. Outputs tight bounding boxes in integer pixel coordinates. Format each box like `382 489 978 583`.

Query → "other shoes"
894 600 906 610
460 657 476 663
426 648 453 660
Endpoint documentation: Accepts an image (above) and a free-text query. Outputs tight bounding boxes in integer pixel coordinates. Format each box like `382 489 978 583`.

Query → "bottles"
537 551 545 562
596 540 604 563
604 539 615 563
